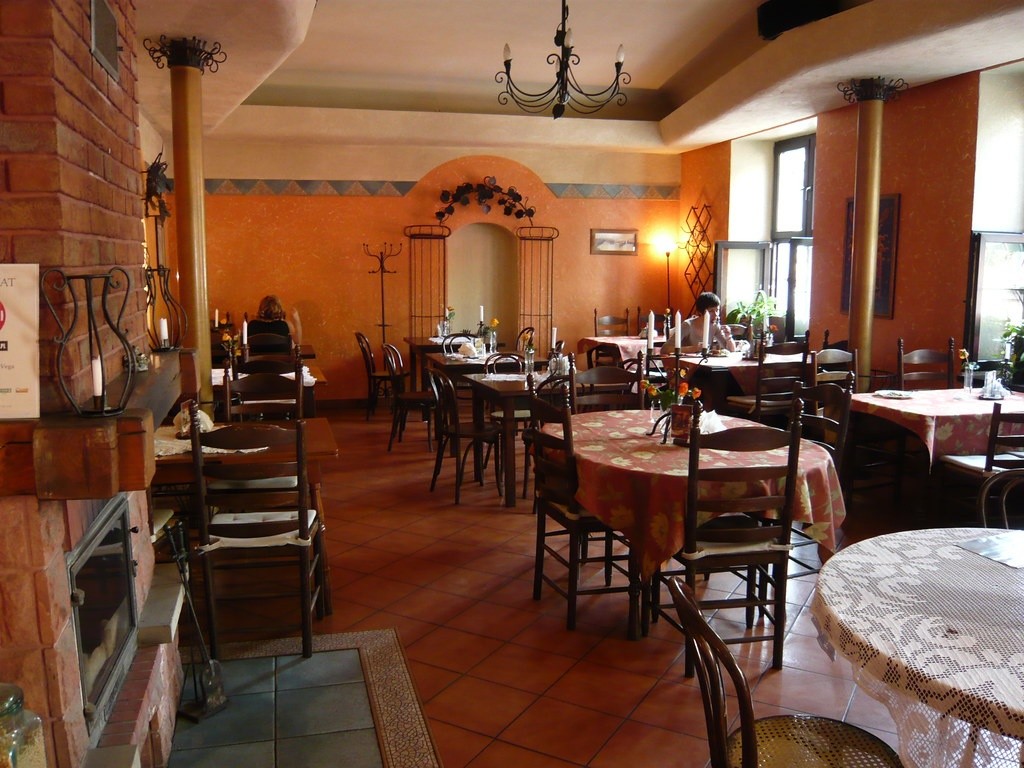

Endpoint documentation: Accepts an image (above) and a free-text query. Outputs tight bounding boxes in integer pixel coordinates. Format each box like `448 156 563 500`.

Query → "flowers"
221 333 242 356
959 348 980 369
447 307 456 334
521 333 535 365
663 308 670 339
487 317 499 333
768 325 777 341
639 369 704 412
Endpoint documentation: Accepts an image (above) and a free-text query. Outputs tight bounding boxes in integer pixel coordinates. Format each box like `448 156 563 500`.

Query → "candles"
1005 343 1010 360
551 327 557 349
92 355 102 396
160 318 168 339
648 310 654 349
702 309 710 348
215 309 218 328
480 306 483 321
446 308 448 317
674 309 681 348
243 321 248 344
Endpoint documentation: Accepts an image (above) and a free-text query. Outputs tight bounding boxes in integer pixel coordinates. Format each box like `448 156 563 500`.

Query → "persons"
660 292 736 356
256 296 301 349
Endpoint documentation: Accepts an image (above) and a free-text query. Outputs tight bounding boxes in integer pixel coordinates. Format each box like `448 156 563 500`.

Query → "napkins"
696 410 729 433
172 411 214 431
459 343 477 357
547 356 577 374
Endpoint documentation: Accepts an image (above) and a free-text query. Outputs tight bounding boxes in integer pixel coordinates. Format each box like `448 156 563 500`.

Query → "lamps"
495 0 631 120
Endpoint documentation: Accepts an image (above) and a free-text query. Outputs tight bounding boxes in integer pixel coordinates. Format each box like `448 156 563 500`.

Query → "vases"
664 320 670 339
490 332 498 354
964 366 973 389
766 334 773 346
659 398 683 435
440 321 453 336
525 350 534 365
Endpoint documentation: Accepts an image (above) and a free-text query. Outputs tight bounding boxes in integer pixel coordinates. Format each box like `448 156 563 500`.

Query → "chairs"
355 306 1024 768
190 334 323 658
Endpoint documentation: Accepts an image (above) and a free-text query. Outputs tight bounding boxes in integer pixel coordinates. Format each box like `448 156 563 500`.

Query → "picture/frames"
840 193 900 319
590 229 639 256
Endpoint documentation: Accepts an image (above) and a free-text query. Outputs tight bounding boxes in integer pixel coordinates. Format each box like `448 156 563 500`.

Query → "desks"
212 345 316 367
424 350 549 457
809 527 1024 768
155 416 340 616
577 335 667 394
211 364 327 419
845 388 1024 528
403 336 506 421
659 351 820 414
460 371 629 507
529 410 846 638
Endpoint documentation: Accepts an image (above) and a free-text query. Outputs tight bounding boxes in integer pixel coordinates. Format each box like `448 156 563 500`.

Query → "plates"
875 389 911 399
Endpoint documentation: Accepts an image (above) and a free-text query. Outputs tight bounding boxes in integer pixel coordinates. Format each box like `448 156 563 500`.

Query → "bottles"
0 681 49 768
